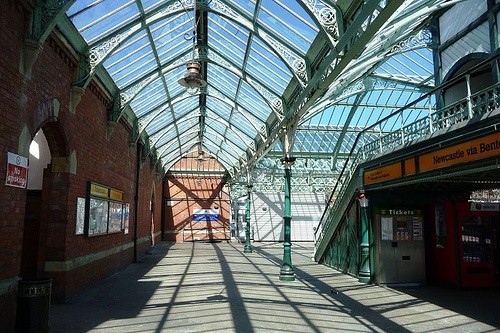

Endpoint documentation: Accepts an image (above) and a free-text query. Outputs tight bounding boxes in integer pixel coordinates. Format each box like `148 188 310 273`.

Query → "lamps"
177 31 208 96
193 139 207 165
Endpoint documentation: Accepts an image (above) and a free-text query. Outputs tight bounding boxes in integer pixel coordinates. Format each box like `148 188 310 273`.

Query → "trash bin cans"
16 276 52 333
